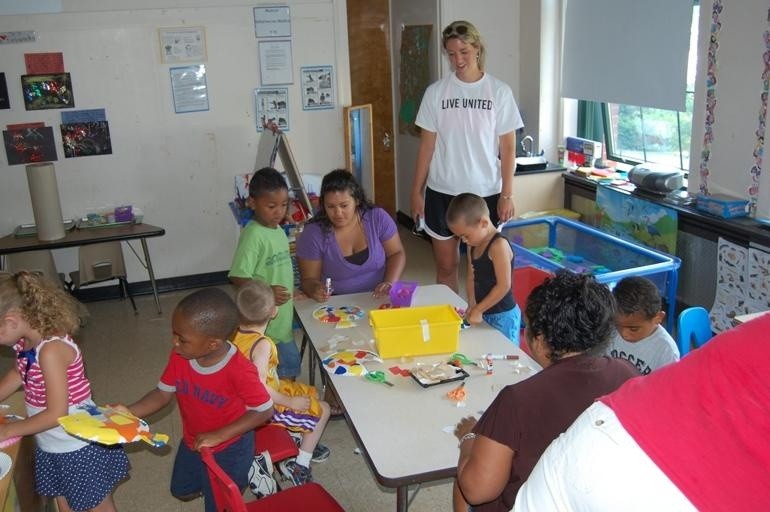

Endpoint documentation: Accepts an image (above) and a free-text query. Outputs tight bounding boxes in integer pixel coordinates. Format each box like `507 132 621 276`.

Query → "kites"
325 277 332 296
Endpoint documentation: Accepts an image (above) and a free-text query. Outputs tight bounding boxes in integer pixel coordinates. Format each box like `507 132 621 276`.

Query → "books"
366 303 465 364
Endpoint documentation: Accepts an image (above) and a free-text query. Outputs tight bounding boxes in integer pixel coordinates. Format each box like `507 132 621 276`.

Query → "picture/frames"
0 390 46 512
0 218 166 316
285 283 559 512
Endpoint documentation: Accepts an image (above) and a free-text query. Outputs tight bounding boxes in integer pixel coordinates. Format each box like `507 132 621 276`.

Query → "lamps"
67 237 139 318
677 306 712 361
196 444 350 512
0 248 73 306
511 263 557 356
250 421 302 492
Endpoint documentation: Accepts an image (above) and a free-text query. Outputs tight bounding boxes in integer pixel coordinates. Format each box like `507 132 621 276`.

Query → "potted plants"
155 25 208 66
168 66 211 116
250 3 338 134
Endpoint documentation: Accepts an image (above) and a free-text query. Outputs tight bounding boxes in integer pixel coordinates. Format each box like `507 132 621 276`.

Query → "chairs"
279 455 314 488
246 449 278 497
299 438 331 462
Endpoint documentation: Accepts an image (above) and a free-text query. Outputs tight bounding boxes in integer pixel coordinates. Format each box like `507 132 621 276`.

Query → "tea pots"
342 103 376 208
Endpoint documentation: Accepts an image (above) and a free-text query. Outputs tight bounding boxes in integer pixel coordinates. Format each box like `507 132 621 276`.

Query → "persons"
227 167 302 382
231 281 330 486
106 288 277 511
446 193 522 346
297 170 407 419
410 20 524 296
607 276 679 376
452 273 644 511
0 270 131 512
510 313 770 511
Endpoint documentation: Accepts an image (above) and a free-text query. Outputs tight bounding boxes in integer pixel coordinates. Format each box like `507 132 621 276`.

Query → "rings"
481 354 519 360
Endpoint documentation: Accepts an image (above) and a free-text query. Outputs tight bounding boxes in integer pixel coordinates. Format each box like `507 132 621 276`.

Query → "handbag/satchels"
382 281 392 286
459 432 475 446
500 195 514 200
